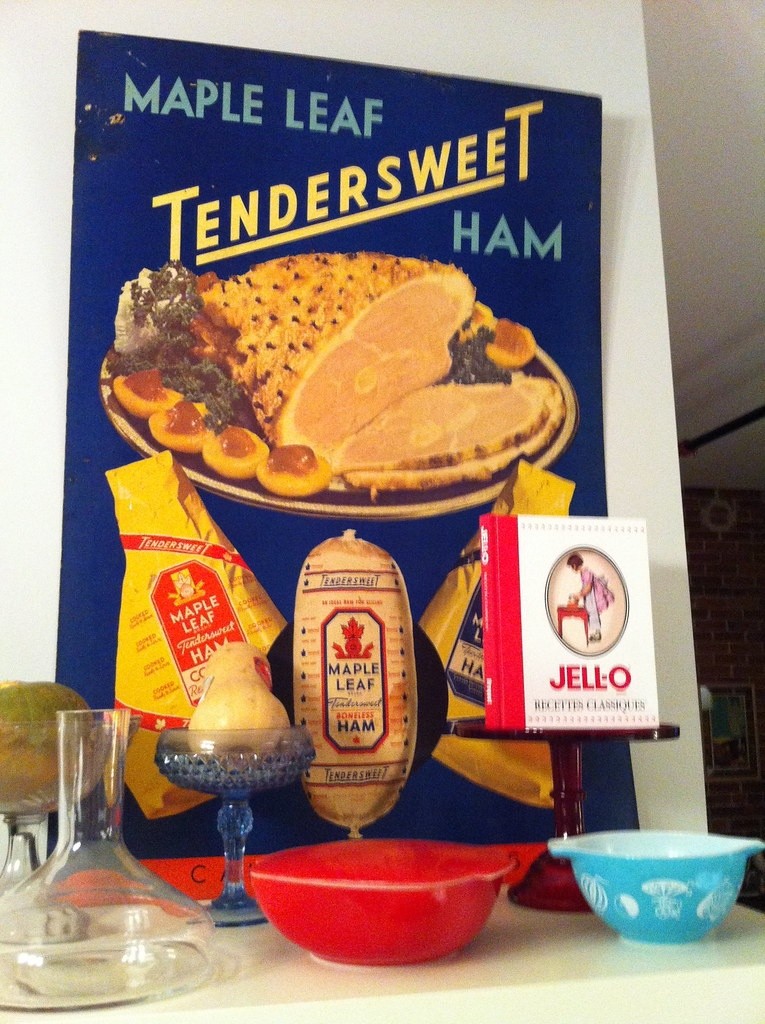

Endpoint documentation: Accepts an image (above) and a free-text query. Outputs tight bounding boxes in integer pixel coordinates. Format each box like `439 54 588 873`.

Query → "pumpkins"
0 680 99 808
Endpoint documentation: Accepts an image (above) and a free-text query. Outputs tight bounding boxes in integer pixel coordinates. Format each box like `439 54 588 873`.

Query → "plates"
95 319 581 523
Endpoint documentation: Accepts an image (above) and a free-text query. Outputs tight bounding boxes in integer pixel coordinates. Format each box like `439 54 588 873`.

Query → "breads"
199 248 566 494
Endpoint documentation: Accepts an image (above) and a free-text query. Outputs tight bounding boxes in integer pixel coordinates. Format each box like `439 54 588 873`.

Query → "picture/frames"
699 682 763 784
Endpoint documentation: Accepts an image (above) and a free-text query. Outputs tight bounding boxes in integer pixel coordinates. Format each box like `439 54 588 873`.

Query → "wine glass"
0 714 143 901
154 724 316 926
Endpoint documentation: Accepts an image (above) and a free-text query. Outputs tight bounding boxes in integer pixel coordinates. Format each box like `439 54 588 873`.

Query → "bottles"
0 708 217 1011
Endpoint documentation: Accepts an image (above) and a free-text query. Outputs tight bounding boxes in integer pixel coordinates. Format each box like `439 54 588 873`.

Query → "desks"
0 884 765 1024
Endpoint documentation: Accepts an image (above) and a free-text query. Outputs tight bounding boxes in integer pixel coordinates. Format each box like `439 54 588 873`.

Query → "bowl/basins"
545 828 765 945
247 837 520 967
